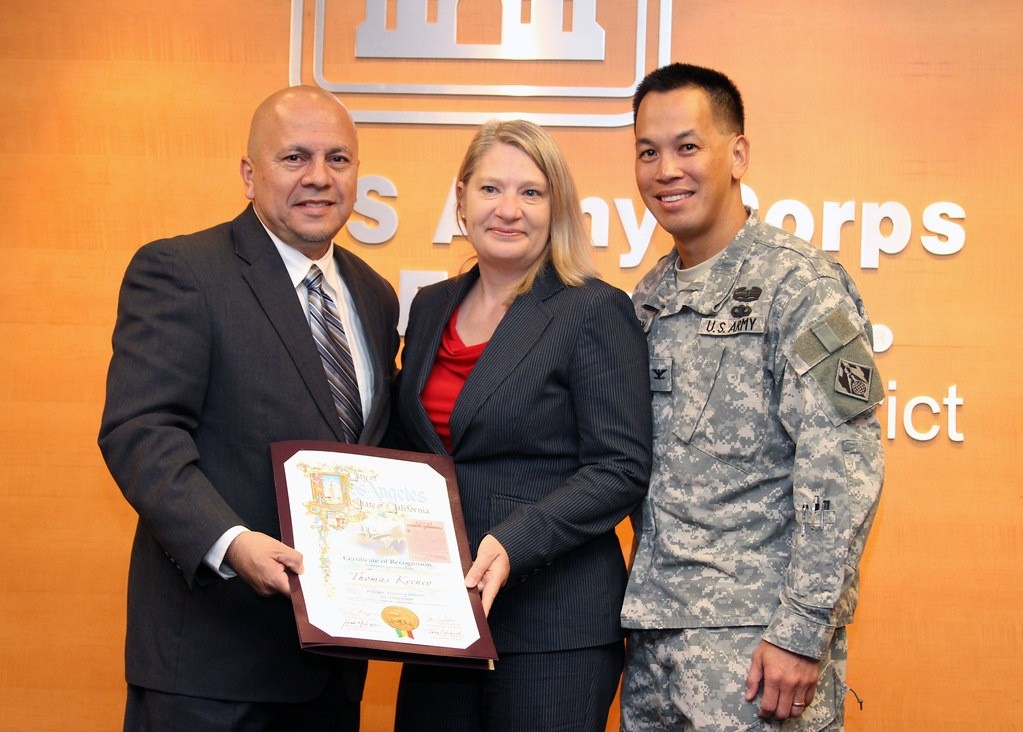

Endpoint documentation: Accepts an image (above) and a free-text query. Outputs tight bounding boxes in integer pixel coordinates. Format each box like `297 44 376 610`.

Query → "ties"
301 266 364 445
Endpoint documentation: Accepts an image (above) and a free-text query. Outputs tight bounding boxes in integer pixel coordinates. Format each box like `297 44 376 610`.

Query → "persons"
617 62 885 731
97 85 400 732
388 121 654 731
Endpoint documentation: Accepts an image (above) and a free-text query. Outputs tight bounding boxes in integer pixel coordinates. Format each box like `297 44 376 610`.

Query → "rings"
792 702 804 706
